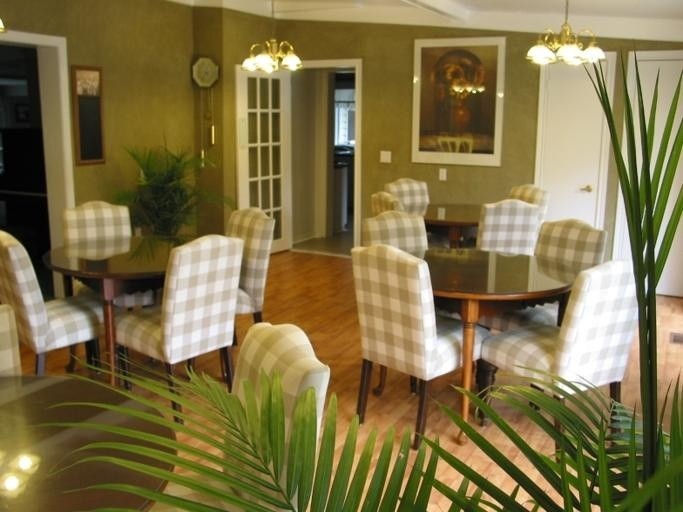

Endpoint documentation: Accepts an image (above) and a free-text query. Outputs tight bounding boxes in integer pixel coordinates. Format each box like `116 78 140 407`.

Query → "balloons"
191 55 220 170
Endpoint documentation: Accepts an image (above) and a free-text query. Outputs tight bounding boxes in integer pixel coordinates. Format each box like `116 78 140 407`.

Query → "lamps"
361 177 609 333
475 259 648 471
160 208 276 345
350 244 492 449
0 231 106 378
60 199 155 311
113 234 245 426
148 321 332 512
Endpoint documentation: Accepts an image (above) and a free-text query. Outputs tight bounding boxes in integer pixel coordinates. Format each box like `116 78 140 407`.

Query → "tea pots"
72 65 107 165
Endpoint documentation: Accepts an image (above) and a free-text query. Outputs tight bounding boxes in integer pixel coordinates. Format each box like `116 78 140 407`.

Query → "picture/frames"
0 377 176 512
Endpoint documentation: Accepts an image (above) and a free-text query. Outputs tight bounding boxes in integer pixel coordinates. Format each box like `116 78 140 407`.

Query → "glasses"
411 36 507 167
240 0 303 73
524 0 606 67
